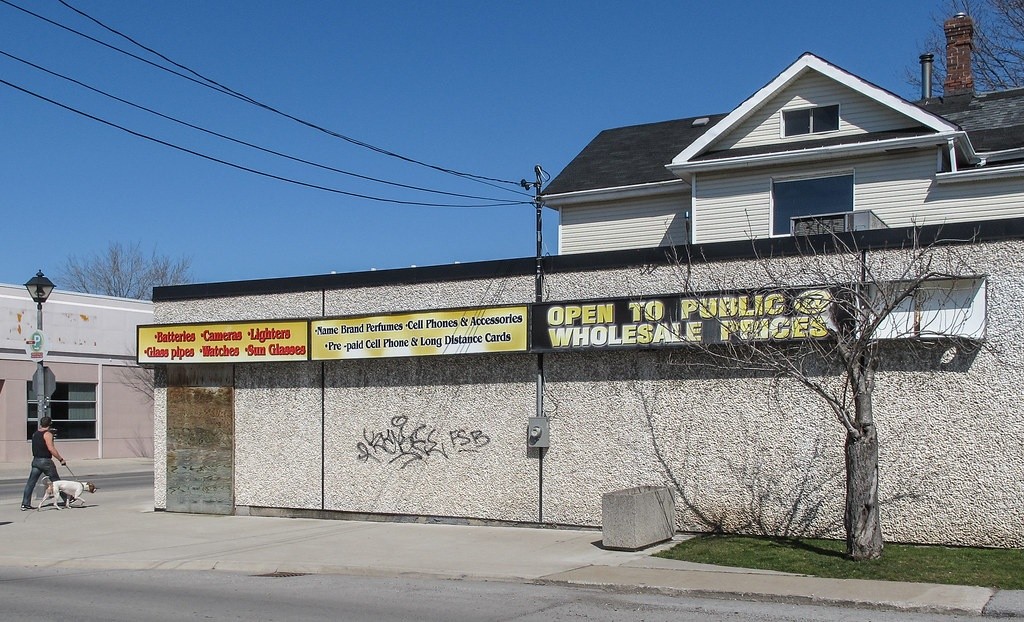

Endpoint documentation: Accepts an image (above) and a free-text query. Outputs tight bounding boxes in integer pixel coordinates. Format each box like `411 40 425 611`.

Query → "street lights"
23 269 57 500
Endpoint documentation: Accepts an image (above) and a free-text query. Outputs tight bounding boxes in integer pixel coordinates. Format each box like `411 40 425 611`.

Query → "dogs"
37 476 97 512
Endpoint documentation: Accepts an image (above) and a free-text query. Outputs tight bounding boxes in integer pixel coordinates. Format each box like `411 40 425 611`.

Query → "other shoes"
21 504 37 511
65 497 78 505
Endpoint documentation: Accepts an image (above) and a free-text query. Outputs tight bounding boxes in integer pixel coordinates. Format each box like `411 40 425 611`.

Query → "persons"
20 416 77 510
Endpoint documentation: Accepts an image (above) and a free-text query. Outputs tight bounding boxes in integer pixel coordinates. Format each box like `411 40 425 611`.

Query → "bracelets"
60 459 63 461
